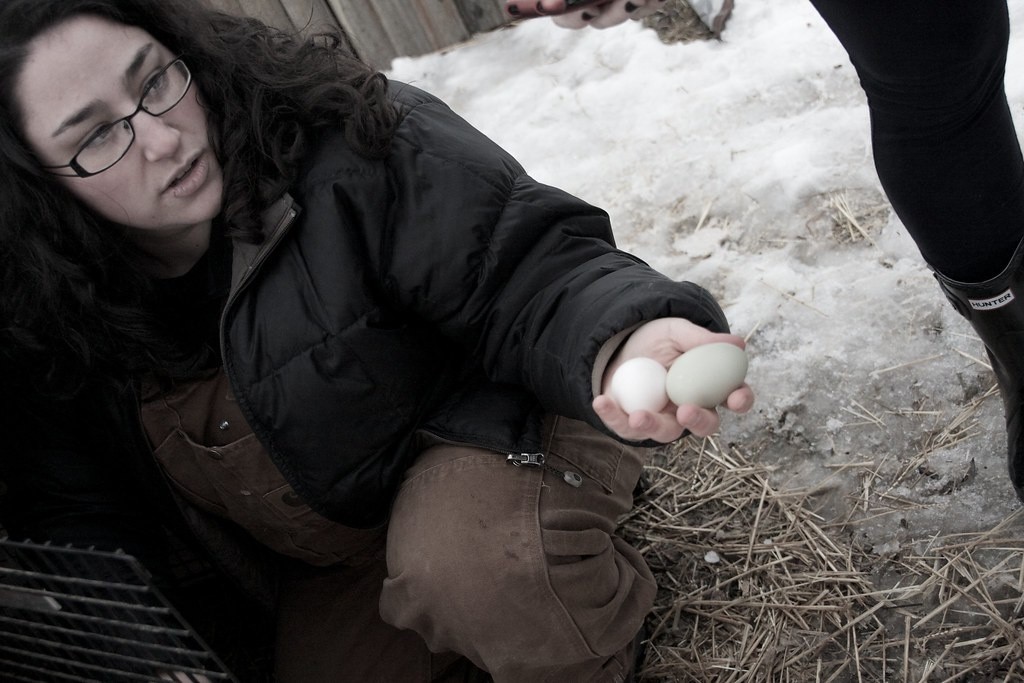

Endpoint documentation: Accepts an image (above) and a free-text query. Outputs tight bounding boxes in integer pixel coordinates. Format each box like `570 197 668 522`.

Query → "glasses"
40 53 192 178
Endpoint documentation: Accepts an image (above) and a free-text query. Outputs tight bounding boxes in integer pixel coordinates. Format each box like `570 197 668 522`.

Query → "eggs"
611 357 670 416
665 343 749 409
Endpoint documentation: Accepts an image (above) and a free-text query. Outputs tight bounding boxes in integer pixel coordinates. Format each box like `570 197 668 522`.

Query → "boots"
926 238 1024 505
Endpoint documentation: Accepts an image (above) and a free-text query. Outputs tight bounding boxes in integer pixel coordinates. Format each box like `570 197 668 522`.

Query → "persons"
0 0 754 683
809 0 1024 505
